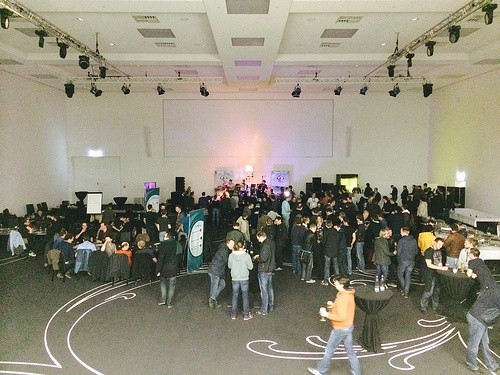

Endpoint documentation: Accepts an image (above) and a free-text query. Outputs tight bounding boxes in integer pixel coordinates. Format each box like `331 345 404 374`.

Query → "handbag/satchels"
299 249 312 264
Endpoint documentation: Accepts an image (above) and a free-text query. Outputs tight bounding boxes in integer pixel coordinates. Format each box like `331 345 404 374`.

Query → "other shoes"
231 315 238 319
470 368 486 375
243 314 254 320
308 367 322 375
208 298 215 310
421 309 430 316
214 302 222 308
158 301 166 305
433 307 442 316
167 304 174 309
306 279 316 284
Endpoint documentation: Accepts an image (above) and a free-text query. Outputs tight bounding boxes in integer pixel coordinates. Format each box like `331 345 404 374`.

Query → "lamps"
482 3 497 25
388 82 400 97
423 80 433 98
335 87 342 96
425 41 436 57
64 80 209 98
291 85 301 97
359 85 367 94
387 65 396 77
448 25 461 43
0 8 107 78
405 53 414 67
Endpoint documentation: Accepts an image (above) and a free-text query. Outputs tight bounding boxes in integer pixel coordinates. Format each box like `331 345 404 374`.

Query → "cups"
44 228 46 233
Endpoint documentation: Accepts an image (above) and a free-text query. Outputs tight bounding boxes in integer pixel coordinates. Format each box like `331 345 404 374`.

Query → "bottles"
375 275 379 292
380 275 385 292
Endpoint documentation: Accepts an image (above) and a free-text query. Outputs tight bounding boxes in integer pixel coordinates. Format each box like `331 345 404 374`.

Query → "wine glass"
319 307 326 321
461 261 465 274
327 301 332 312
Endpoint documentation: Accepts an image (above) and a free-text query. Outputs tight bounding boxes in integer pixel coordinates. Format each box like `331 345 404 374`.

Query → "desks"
75 191 89 200
436 224 500 262
437 269 481 323
356 285 394 354
114 197 128 206
30 228 47 236
449 207 500 235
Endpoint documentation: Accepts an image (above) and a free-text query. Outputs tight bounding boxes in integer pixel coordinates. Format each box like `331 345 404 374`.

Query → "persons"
44 203 186 279
371 183 479 314
155 229 183 308
465 248 500 375
19 210 57 256
173 180 383 287
208 230 276 321
307 276 362 375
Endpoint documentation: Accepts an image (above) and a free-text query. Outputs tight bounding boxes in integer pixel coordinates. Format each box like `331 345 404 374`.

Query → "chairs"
7 201 155 286
